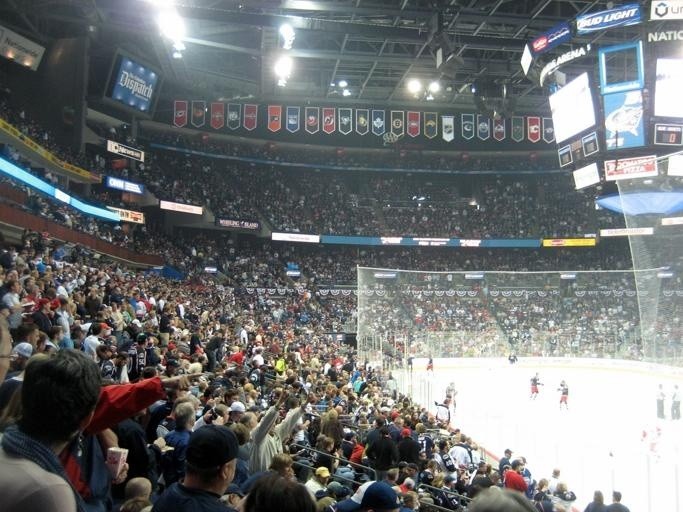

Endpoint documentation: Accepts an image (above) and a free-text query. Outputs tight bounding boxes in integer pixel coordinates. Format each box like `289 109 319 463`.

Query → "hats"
5 248 358 505
363 374 525 490
